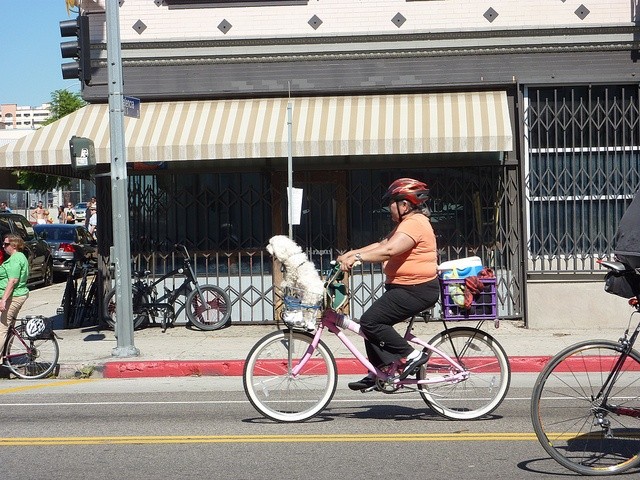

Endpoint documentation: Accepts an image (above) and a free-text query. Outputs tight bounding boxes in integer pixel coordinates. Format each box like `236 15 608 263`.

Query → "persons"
31 201 47 225
337 178 441 390
88 207 97 242
0 202 12 214
90 196 97 209
85 202 92 228
613 187 640 313
57 206 65 224
0 234 30 379
65 202 77 223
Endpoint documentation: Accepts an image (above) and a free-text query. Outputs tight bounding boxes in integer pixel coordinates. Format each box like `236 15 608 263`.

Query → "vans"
71 202 88 222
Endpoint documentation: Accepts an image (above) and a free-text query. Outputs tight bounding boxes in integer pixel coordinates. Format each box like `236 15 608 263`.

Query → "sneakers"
348 377 386 391
399 351 429 381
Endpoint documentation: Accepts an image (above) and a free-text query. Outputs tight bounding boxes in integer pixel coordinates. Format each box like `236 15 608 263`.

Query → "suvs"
0 213 54 288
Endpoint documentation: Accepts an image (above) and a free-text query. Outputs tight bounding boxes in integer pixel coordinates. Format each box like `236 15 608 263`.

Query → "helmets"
382 177 430 209
25 318 46 338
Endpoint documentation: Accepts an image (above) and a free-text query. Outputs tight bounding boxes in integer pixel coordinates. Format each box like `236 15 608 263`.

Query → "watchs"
355 253 364 263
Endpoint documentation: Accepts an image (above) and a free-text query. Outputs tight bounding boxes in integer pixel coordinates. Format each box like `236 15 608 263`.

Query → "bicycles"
100 243 232 332
529 258 640 477
243 257 512 423
69 266 95 328
0 314 64 379
50 257 78 328
78 272 99 328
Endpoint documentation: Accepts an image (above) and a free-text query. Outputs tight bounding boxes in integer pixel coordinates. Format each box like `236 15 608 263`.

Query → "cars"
32 224 98 279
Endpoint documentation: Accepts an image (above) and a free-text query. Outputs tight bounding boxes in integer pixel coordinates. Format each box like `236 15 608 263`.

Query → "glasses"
2 243 11 248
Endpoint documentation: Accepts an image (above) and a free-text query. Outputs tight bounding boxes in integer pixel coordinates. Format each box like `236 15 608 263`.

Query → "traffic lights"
58 15 93 86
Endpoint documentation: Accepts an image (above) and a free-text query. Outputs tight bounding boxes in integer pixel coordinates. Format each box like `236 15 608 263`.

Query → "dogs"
265 235 325 329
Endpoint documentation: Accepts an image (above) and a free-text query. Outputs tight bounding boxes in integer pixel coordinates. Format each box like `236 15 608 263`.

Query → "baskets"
21 318 53 340
284 285 324 329
